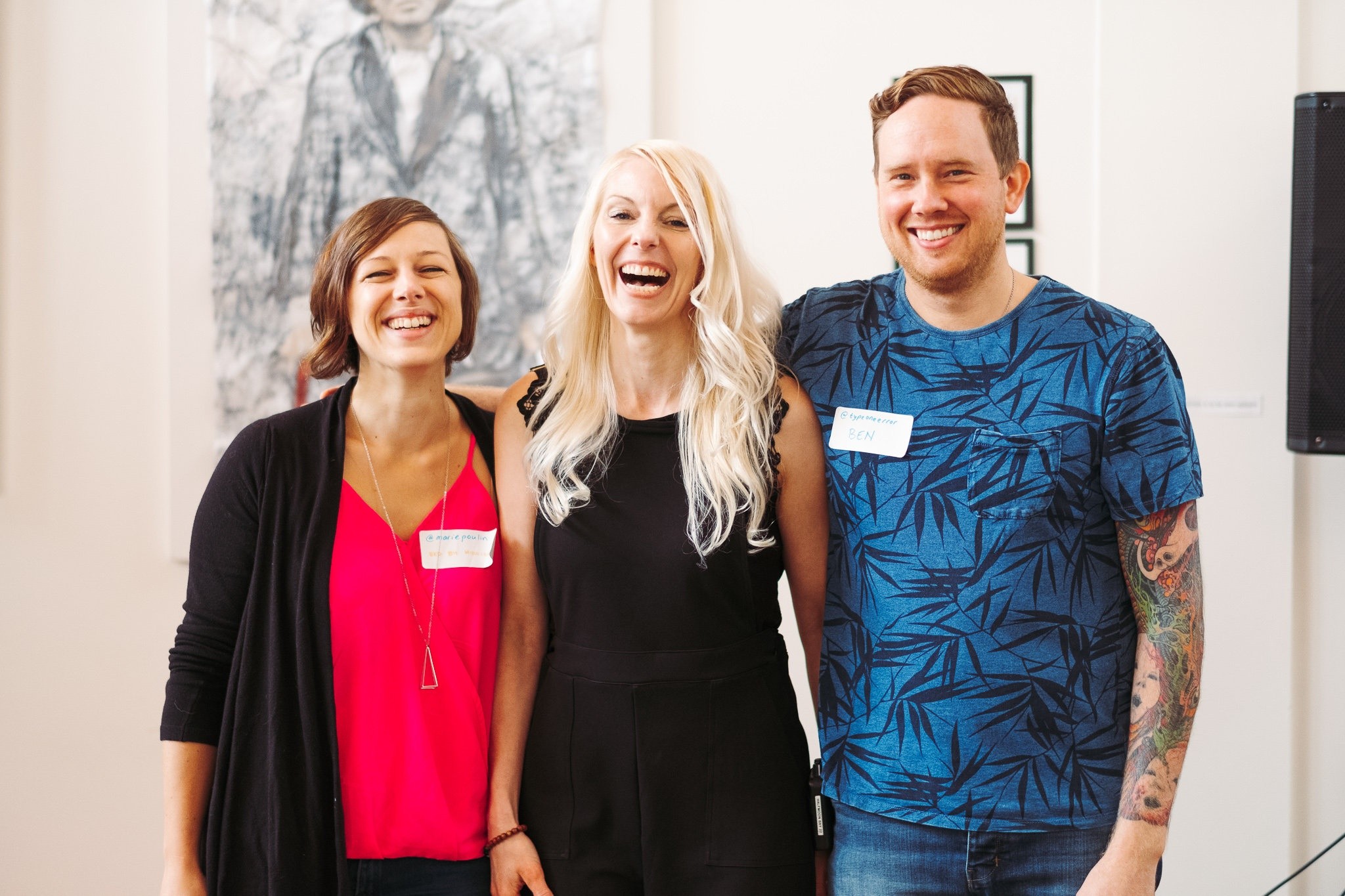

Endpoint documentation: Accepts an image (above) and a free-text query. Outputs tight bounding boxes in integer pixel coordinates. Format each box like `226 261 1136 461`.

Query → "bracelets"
484 826 527 857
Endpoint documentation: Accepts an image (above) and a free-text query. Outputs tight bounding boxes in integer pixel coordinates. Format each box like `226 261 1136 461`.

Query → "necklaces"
348 399 451 689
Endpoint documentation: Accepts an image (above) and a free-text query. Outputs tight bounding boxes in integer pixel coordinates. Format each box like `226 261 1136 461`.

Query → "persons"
486 144 828 896
323 71 1202 896
161 197 504 896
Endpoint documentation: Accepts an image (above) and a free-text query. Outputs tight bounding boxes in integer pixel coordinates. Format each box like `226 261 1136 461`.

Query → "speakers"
1288 90 1344 454
